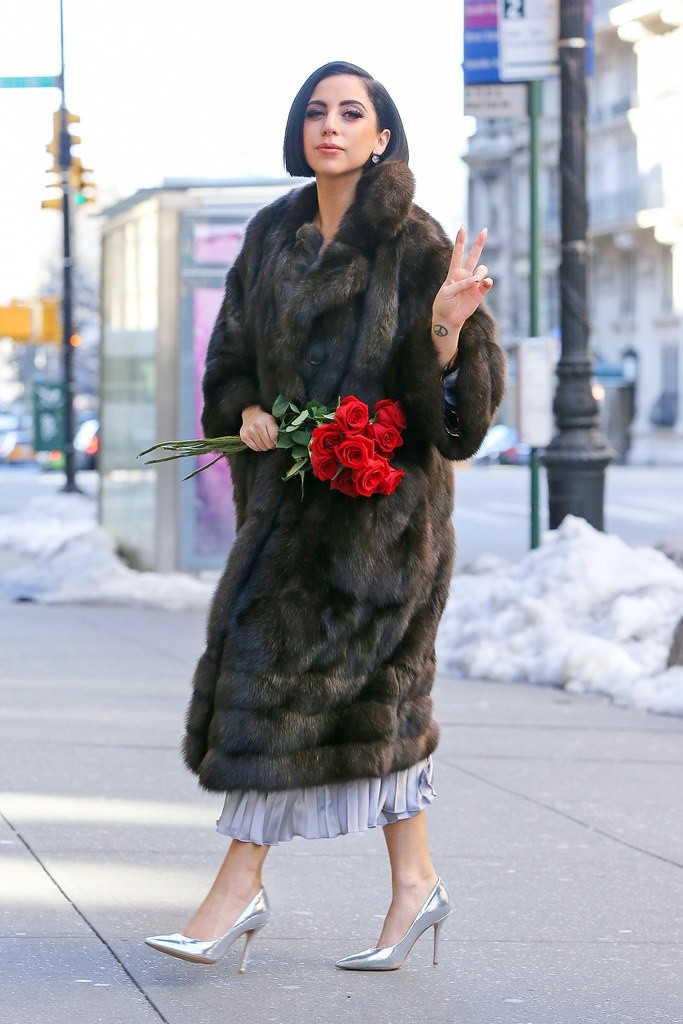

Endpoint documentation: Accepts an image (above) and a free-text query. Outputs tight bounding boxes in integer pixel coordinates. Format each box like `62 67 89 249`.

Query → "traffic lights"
39 165 66 211
66 155 98 211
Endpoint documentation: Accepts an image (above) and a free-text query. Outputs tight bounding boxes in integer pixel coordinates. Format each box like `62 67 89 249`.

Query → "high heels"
334 879 451 972
145 884 269 974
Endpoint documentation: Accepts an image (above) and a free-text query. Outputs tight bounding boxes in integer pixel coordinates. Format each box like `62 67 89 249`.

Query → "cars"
1 401 102 472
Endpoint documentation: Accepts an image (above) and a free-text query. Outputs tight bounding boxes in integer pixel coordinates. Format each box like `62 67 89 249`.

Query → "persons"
144 60 507 973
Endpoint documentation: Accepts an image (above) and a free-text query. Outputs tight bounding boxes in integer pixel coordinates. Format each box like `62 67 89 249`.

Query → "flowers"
136 393 406 500
